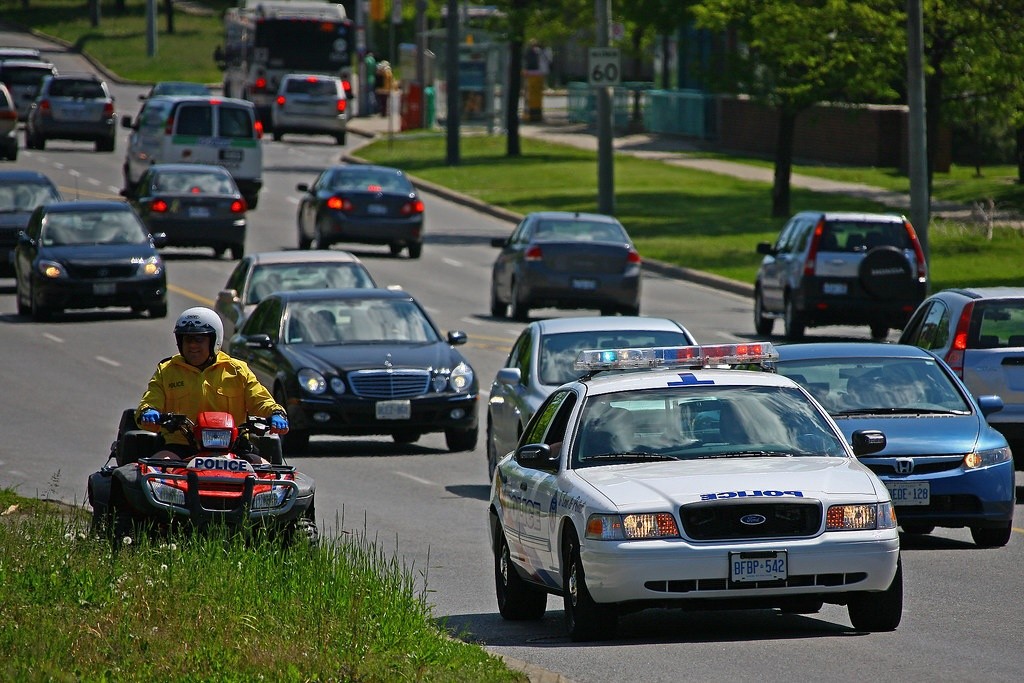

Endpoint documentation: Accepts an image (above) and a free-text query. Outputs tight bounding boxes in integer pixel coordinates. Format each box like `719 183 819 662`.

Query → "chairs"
846 233 862 247
584 433 611 455
720 402 752 443
1008 335 1024 346
312 310 341 341
980 335 999 346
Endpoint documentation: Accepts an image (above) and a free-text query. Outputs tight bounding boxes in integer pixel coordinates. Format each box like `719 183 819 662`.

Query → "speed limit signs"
590 46 627 90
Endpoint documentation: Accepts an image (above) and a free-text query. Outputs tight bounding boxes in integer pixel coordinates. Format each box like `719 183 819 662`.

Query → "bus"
210 0 361 129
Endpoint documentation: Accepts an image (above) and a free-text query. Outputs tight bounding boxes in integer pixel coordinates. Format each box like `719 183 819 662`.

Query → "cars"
137 82 216 101
895 287 1024 445
0 45 42 63
270 75 354 145
295 164 426 258
120 161 249 258
0 169 65 269
211 250 404 355
0 84 23 157
11 197 171 321
0 59 59 123
478 317 706 484
484 342 908 638
489 211 645 317
729 341 1016 549
228 289 482 453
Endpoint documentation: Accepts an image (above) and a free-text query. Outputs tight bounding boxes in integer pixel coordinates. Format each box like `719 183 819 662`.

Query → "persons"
521 38 548 122
362 306 399 340
46 221 79 243
583 408 639 455
317 269 343 289
372 60 401 117
266 273 286 292
135 307 290 473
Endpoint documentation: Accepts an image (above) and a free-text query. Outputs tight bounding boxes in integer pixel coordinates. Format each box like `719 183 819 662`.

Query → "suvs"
753 209 935 341
25 72 121 153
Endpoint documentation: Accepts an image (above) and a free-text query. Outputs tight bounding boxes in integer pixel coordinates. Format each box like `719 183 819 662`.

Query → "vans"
116 95 264 212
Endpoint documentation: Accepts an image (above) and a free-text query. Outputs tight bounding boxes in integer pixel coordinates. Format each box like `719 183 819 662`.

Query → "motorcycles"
91 404 319 547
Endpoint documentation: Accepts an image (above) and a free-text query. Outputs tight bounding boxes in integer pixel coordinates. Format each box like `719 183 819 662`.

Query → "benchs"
817 233 883 249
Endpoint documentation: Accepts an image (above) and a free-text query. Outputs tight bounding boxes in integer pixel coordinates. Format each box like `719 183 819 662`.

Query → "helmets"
173 307 223 357
